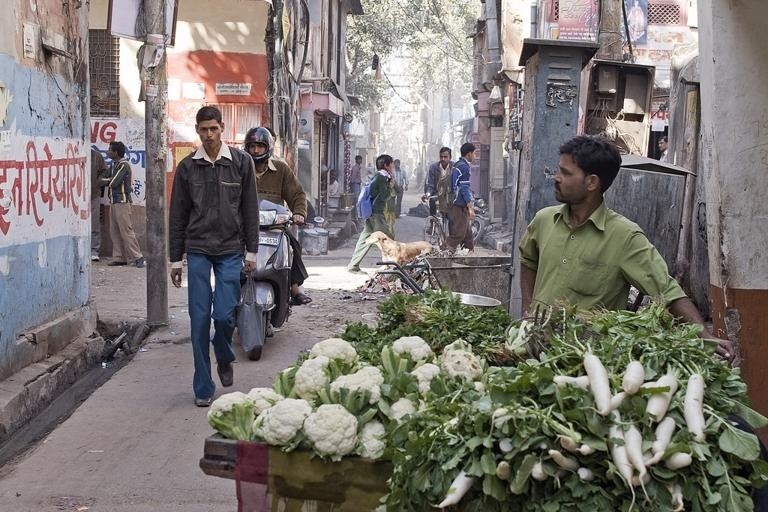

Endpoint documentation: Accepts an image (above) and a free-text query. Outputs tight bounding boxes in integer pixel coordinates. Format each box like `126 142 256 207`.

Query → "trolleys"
376 253 512 319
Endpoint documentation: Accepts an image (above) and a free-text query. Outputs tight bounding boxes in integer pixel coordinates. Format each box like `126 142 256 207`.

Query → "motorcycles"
240 198 307 359
468 191 492 244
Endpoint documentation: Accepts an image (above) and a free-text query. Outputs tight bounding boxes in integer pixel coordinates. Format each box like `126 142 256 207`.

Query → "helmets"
243 127 275 161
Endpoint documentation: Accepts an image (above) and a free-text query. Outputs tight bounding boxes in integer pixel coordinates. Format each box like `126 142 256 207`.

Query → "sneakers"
249 345 262 360
91 253 99 261
293 292 311 304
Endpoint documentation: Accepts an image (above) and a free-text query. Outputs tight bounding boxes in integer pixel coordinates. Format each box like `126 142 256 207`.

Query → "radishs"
429 354 706 512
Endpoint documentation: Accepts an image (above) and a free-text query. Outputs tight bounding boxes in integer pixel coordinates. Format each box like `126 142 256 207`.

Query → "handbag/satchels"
236 273 265 352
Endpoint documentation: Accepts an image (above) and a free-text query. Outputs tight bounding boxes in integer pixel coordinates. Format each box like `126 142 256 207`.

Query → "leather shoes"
217 362 233 387
136 257 144 268
108 259 128 266
195 393 212 407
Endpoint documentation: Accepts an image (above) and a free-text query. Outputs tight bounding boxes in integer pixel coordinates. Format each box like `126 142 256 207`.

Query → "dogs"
365 230 436 262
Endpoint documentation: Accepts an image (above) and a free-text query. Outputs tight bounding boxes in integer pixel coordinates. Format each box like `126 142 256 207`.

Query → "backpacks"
357 174 381 219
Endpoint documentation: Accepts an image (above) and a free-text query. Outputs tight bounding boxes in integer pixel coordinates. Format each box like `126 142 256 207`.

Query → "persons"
244 127 313 305
658 136 669 162
441 143 476 253
346 155 396 275
168 106 260 407
97 141 144 268
413 163 424 189
329 169 339 196
350 155 362 217
518 134 736 365
393 159 409 219
626 0 645 43
421 147 455 235
91 149 107 261
266 127 299 243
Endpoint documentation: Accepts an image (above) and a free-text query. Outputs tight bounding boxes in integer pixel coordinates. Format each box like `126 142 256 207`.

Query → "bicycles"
421 191 449 253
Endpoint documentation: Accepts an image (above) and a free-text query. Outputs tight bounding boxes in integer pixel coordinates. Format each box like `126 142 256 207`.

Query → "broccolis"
207 336 485 463
